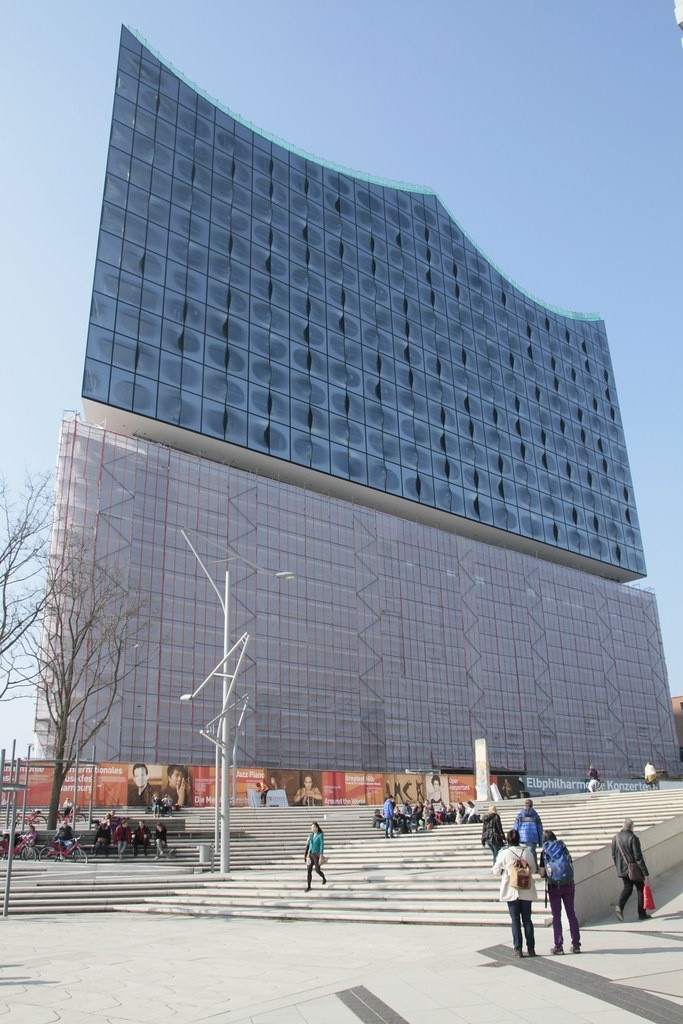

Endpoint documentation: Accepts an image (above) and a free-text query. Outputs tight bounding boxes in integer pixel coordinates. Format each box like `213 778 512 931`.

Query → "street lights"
180 528 298 875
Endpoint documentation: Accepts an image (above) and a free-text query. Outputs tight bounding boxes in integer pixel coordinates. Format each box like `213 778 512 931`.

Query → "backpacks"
506 847 531 889
543 840 574 885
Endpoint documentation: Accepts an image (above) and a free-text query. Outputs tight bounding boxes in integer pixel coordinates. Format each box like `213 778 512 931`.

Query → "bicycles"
38 833 90 864
0 833 37 861
8 810 47 826
47 807 86 823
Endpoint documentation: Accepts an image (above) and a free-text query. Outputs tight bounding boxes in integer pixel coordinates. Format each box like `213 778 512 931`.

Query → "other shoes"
640 914 651 920
305 887 311 892
550 945 564 955
322 878 326 884
529 951 536 956
616 905 624 921
570 945 580 953
512 951 523 958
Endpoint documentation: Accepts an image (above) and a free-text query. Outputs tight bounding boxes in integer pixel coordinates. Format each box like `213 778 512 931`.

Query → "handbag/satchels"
318 856 327 865
629 862 645 881
644 885 655 910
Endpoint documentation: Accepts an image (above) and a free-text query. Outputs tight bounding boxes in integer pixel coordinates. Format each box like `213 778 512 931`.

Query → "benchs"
8 813 86 826
17 844 166 854
91 818 99 828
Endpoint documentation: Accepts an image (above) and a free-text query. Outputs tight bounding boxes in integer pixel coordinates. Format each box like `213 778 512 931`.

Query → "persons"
127 763 192 808
492 830 538 958
304 821 326 892
373 795 545 875
612 818 652 922
270 772 322 806
385 775 445 805
644 761 657 791
501 778 513 799
588 764 600 793
22 794 173 862
540 830 582 954
